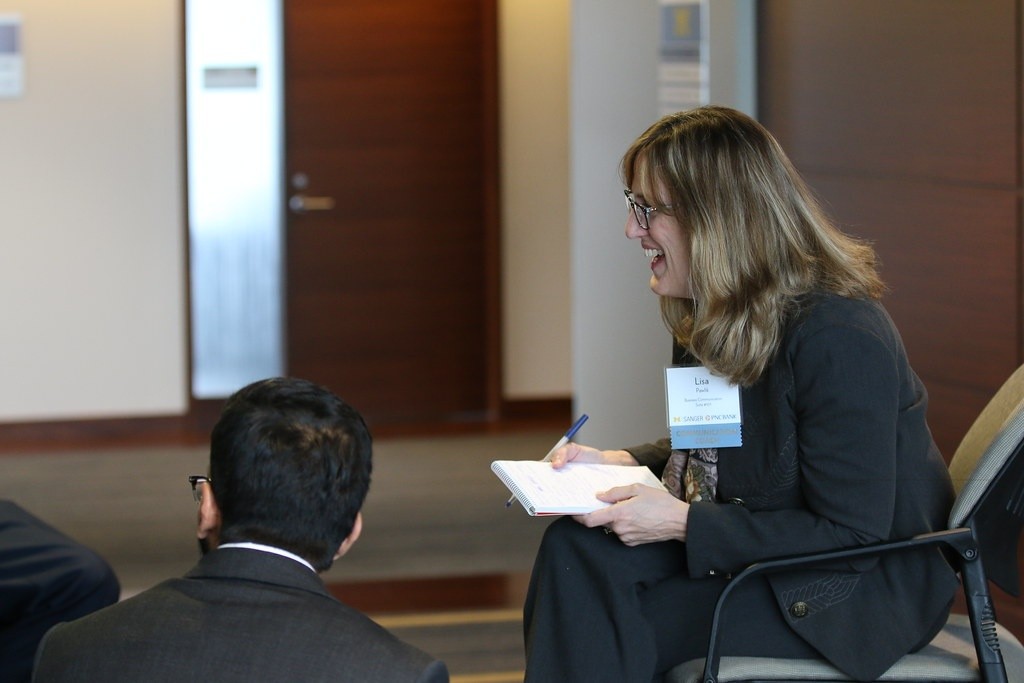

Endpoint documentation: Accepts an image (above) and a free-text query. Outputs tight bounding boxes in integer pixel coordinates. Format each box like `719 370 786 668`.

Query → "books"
490 461 668 517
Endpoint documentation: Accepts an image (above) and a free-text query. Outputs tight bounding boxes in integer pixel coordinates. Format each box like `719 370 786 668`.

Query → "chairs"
664 361 1024 683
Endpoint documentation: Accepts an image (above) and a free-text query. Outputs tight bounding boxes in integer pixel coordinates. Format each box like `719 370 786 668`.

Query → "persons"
522 108 959 683
32 377 451 683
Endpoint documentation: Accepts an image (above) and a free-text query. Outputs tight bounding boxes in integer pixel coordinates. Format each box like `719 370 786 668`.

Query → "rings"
604 528 612 534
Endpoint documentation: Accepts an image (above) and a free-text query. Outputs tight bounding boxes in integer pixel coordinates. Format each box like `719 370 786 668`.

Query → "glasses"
624 189 681 230
188 475 211 504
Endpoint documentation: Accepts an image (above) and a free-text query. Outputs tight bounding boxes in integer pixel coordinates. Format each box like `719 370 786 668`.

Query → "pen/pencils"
505 414 589 508
